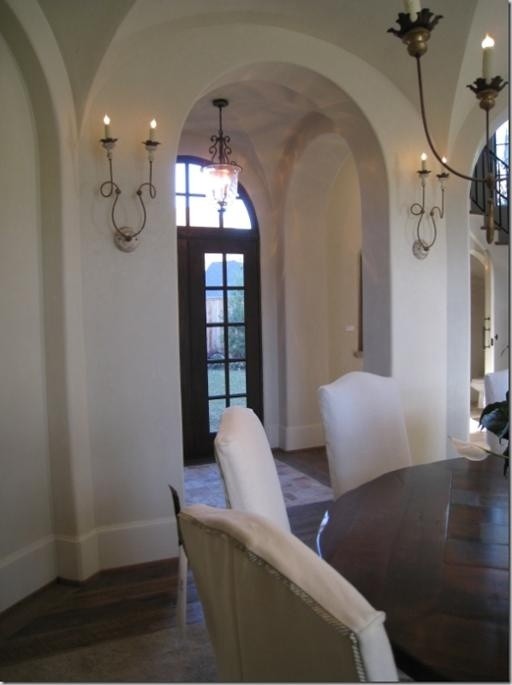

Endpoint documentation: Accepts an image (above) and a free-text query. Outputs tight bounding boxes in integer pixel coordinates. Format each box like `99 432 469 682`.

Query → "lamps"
389 0 511 248
202 93 242 217
91 107 164 258
407 151 450 259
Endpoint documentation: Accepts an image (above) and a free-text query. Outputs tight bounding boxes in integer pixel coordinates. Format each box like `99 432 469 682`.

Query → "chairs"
483 365 508 459
209 401 292 532
316 369 410 498
175 497 405 685
